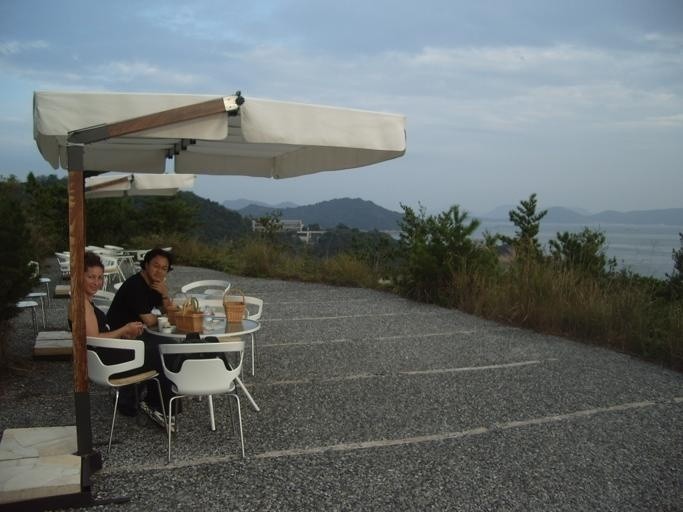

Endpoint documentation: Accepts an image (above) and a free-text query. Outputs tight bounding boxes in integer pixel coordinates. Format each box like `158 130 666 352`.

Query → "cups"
162 327 172 333
157 316 168 332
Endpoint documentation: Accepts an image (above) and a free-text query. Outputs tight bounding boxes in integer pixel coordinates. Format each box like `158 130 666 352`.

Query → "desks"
145 311 261 431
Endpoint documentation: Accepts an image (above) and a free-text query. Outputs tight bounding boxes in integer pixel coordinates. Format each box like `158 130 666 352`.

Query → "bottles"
204 305 213 335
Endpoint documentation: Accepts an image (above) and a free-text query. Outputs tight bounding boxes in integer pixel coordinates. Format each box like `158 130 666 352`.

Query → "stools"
40 277 51 305
15 301 39 338
27 293 47 328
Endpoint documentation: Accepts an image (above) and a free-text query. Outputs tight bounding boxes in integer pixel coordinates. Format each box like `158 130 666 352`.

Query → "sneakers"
139 401 179 433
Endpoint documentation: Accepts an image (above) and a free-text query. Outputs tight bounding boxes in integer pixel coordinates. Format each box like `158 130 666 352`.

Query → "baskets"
167 305 205 333
222 287 246 323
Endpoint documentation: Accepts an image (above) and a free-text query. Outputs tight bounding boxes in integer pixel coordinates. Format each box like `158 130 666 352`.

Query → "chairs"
157 341 246 463
181 279 232 298
196 296 263 377
56 244 173 296
86 336 168 454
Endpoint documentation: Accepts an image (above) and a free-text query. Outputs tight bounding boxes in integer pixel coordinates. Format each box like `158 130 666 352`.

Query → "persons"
101 247 174 417
67 250 183 433
